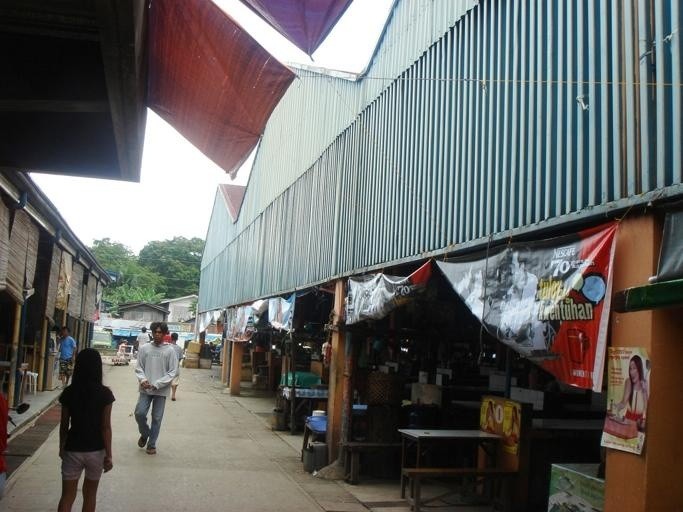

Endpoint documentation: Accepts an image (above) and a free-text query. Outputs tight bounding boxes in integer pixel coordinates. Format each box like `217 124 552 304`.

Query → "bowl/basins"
307 415 327 432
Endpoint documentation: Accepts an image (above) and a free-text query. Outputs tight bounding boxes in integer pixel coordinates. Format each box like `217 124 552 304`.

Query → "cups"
566 329 586 363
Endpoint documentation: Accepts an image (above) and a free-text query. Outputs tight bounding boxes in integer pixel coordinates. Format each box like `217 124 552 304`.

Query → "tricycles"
110 353 130 366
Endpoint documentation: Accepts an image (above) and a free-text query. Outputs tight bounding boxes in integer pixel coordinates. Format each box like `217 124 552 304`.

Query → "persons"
482 401 495 431
163 331 170 342
119 339 128 353
58 325 76 391
613 354 647 431
135 326 150 350
168 333 183 402
133 321 179 455
55 347 116 511
0 368 9 495
506 406 519 440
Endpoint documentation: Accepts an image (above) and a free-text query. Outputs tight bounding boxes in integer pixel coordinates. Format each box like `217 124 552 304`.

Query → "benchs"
341 440 503 510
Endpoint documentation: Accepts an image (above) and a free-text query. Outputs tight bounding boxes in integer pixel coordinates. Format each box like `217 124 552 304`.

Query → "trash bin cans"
271 409 286 431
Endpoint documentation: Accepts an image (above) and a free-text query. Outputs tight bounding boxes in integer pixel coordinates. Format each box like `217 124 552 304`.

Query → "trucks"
91 330 113 353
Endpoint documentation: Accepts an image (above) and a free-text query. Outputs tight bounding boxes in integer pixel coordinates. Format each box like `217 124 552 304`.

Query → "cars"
210 343 223 365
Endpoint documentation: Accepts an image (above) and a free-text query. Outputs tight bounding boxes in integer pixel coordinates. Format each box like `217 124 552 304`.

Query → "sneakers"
137 436 146 448
172 398 176 401
145 448 157 455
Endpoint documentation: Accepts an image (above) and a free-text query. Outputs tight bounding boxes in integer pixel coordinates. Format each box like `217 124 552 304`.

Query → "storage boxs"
183 340 212 370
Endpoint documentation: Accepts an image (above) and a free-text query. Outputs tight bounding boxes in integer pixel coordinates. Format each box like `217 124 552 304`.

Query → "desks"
396 420 503 510
